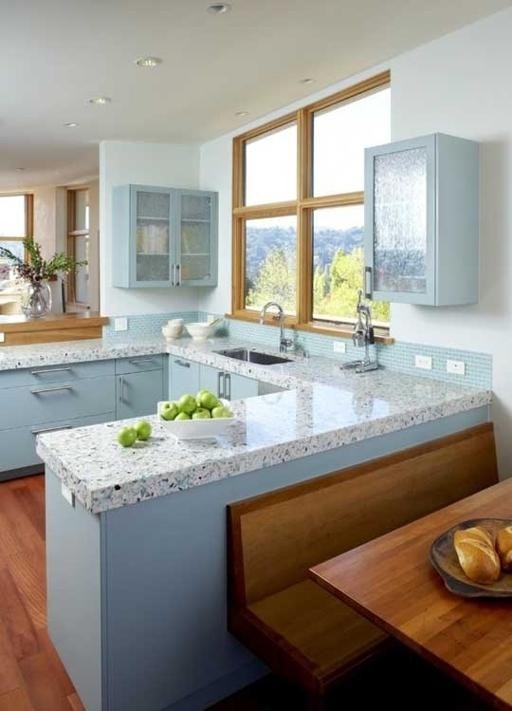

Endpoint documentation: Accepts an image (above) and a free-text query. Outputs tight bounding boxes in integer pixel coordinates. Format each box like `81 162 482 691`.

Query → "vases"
21 281 52 318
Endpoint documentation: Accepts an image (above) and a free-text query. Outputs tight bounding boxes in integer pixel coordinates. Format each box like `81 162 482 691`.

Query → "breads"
495 524 512 570
456 526 500 584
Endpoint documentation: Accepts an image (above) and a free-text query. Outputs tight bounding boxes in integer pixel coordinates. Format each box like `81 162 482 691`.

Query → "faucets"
257 302 288 347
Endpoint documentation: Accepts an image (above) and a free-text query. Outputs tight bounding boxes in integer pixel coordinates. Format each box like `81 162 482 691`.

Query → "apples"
118 426 137 447
160 390 229 421
134 420 152 440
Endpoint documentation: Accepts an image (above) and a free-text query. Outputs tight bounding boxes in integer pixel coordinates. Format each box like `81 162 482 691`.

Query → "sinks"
211 346 295 366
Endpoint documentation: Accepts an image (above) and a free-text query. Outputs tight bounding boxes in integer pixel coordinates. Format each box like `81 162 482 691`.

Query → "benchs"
227 421 498 710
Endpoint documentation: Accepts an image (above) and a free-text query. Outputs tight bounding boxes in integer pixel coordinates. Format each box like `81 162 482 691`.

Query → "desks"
0 312 109 346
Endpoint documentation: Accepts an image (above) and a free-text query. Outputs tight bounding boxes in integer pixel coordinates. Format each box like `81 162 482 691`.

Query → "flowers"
1 239 88 281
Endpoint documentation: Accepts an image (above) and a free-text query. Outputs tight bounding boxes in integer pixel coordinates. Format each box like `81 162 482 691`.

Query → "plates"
429 517 511 599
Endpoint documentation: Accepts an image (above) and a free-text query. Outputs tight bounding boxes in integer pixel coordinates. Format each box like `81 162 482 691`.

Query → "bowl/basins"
160 324 183 341
185 321 216 340
155 396 239 440
167 318 184 338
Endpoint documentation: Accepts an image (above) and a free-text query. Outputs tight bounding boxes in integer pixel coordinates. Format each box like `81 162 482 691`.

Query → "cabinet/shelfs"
168 353 287 402
0 355 167 481
36 407 490 710
364 133 481 307
113 183 218 288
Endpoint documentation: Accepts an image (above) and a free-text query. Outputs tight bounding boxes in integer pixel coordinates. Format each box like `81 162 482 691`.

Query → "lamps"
137 57 162 68
89 96 111 105
